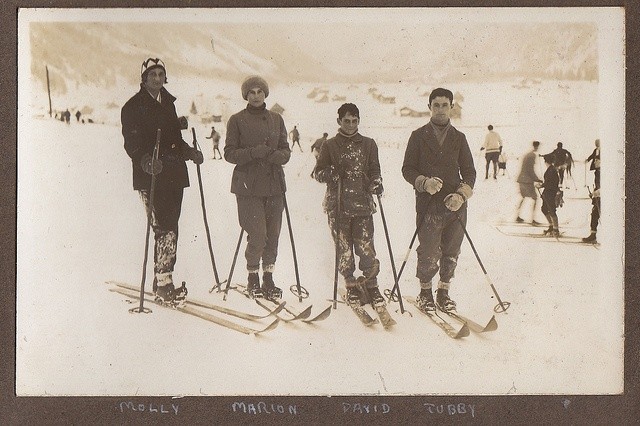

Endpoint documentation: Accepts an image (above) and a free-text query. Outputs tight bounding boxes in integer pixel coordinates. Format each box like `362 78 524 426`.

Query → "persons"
480 125 503 179
585 140 600 171
517 141 543 226
224 73 291 299
205 127 222 159
311 133 328 179
538 155 560 234
401 87 477 316
583 155 600 242
538 142 575 184
53 109 98 126
121 56 204 309
314 104 387 307
289 125 303 153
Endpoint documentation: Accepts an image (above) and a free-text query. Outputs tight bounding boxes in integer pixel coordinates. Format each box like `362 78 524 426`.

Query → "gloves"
265 150 284 165
249 141 271 159
414 175 443 196
499 146 502 151
368 176 384 195
480 148 484 151
186 147 204 164
140 154 164 176
444 182 474 212
327 168 340 184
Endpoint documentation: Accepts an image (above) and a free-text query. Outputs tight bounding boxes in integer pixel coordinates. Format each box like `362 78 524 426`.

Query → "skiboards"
231 282 332 322
402 293 497 339
544 239 600 247
336 283 398 328
496 227 579 238
104 279 287 335
499 220 570 227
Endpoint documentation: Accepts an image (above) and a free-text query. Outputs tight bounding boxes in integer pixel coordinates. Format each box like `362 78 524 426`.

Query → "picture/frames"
1 0 640 426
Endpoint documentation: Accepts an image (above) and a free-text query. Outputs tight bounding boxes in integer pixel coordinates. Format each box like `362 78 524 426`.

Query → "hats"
241 73 270 101
140 56 167 77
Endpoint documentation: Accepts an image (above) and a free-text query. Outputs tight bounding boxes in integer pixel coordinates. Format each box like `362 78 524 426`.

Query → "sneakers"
550 229 559 234
345 277 362 299
544 227 552 233
262 272 274 289
247 273 260 291
153 278 158 291
365 279 386 304
582 233 597 242
437 289 456 309
532 220 541 225
419 288 435 310
516 217 524 221
157 283 175 303
